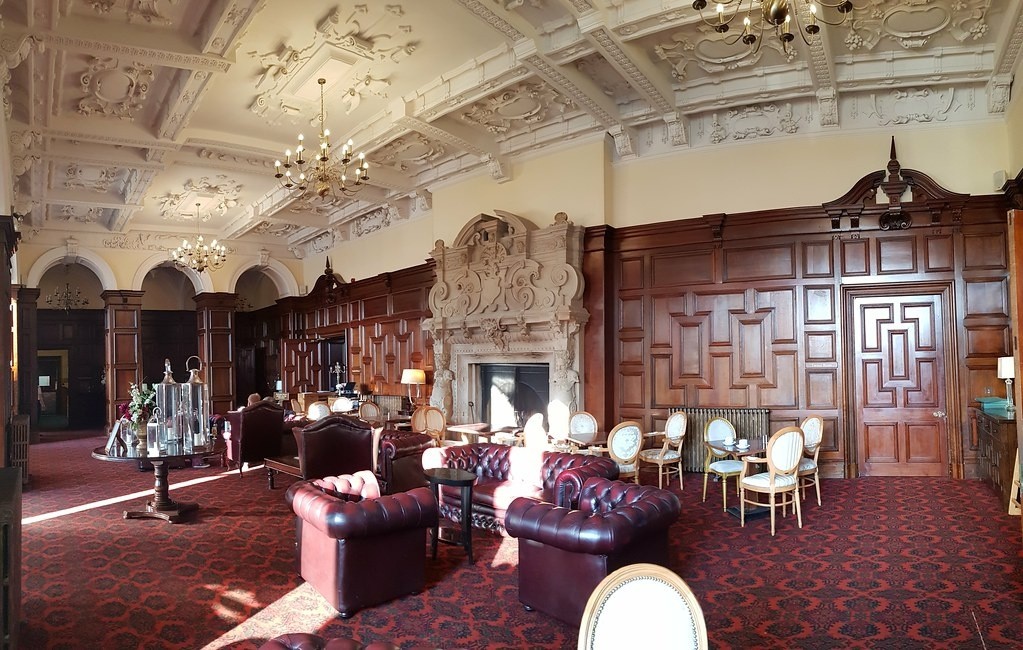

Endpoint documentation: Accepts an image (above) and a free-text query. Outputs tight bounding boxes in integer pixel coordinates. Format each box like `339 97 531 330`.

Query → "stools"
422 468 478 564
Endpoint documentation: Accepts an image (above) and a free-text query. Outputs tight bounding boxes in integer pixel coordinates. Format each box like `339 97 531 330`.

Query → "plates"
736 445 750 449
722 441 736 445
737 448 749 452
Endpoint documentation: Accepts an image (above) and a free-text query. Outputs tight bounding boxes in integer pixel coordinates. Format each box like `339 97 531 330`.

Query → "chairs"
248 392 823 536
577 563 709 650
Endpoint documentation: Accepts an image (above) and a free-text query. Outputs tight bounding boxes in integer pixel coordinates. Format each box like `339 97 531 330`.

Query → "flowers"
118 376 157 430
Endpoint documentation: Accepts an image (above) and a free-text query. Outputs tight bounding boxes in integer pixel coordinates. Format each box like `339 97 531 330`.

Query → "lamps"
275 77 369 202
172 203 226 275
401 369 426 404
234 288 254 314
998 356 1015 419
691 0 852 54
45 278 89 316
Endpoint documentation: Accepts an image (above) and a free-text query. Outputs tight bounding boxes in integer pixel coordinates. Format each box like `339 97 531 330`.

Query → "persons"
429 353 455 421
549 352 580 414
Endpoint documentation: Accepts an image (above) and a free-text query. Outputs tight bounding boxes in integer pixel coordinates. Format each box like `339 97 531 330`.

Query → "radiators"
371 394 408 416
10 413 28 484
668 406 770 472
0 466 23 650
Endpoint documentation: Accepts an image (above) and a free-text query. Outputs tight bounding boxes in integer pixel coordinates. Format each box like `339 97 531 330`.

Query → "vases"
133 420 149 450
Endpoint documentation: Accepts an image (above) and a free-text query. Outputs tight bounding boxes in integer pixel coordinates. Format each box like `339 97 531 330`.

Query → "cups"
725 436 733 443
726 446 734 452
739 439 747 447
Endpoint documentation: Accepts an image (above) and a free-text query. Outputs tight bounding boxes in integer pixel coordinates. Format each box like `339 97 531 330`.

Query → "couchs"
224 403 285 477
422 443 619 537
166 414 225 437
293 415 437 496
506 478 681 629
284 470 439 620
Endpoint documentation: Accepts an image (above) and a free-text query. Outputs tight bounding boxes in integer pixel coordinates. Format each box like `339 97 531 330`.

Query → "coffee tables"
262 454 303 489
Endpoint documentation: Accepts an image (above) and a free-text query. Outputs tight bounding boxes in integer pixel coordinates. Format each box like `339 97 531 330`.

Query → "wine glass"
212 427 218 439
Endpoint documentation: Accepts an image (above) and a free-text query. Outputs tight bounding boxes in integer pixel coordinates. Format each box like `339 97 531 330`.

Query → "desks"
564 432 608 445
974 408 1017 512
92 435 227 523
704 438 770 520
360 416 411 429
447 422 523 443
